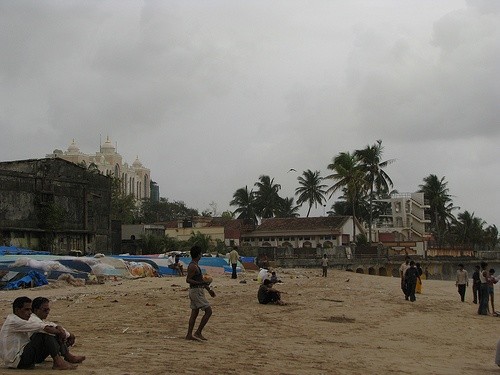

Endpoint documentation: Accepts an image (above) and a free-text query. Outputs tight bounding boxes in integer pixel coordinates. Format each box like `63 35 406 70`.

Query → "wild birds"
287 169 296 173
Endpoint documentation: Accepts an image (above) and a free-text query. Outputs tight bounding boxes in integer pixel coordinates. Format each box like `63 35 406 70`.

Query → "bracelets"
208 288 211 292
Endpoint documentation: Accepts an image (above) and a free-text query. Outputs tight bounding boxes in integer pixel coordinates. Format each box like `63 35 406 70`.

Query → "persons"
320 254 329 277
456 264 469 303
27 297 86 364
0 296 79 370
201 249 233 258
164 252 190 276
185 246 216 342
472 263 498 315
399 256 422 302
229 247 239 279
257 267 282 305
